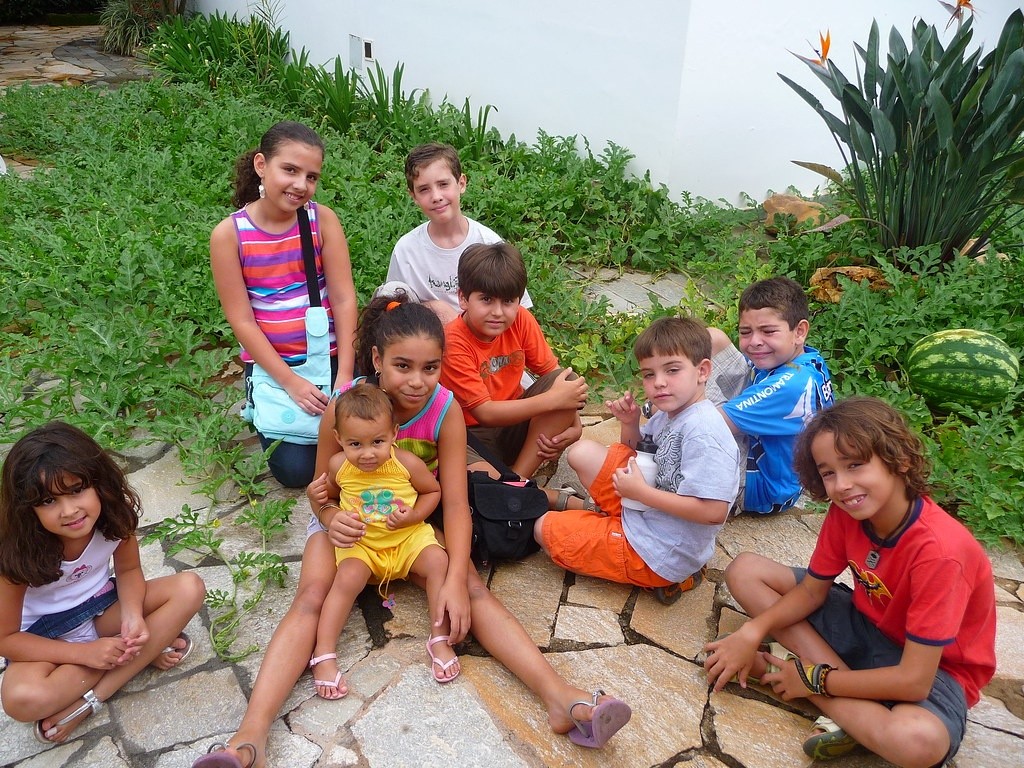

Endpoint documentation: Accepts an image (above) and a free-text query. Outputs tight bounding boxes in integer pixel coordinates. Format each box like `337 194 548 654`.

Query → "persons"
0 420 207 744
651 277 835 517
703 396 997 768
209 121 359 489
385 143 534 326
441 242 606 513
535 316 740 607
307 383 461 700
193 293 632 768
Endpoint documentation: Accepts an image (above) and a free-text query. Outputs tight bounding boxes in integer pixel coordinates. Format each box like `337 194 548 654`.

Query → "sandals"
556 481 601 515
308 653 348 700
426 633 460 683
162 632 194 667
34 689 105 744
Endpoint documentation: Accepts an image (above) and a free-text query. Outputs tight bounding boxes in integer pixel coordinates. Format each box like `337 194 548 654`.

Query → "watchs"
643 401 653 419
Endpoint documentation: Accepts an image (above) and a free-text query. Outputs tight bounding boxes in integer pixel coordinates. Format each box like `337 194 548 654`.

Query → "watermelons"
902 328 1019 415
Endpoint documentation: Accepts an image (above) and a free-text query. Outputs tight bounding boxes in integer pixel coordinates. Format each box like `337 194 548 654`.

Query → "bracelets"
795 658 838 698
318 503 341 534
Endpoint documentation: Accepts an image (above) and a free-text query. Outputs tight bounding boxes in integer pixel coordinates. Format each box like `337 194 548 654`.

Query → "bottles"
620 434 658 512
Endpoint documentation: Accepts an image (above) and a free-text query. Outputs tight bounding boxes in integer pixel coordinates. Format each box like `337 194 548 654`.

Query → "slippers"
568 688 632 749
191 741 256 768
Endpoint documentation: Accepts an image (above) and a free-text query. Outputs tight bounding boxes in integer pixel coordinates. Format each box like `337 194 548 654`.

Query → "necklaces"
865 498 914 570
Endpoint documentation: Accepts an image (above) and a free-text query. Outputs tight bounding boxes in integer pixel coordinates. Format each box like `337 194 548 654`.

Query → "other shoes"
707 632 800 687
655 564 707 606
802 716 874 762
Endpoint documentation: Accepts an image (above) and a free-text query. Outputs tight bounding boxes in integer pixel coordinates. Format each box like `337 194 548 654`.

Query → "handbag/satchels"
240 306 332 444
466 469 549 563
